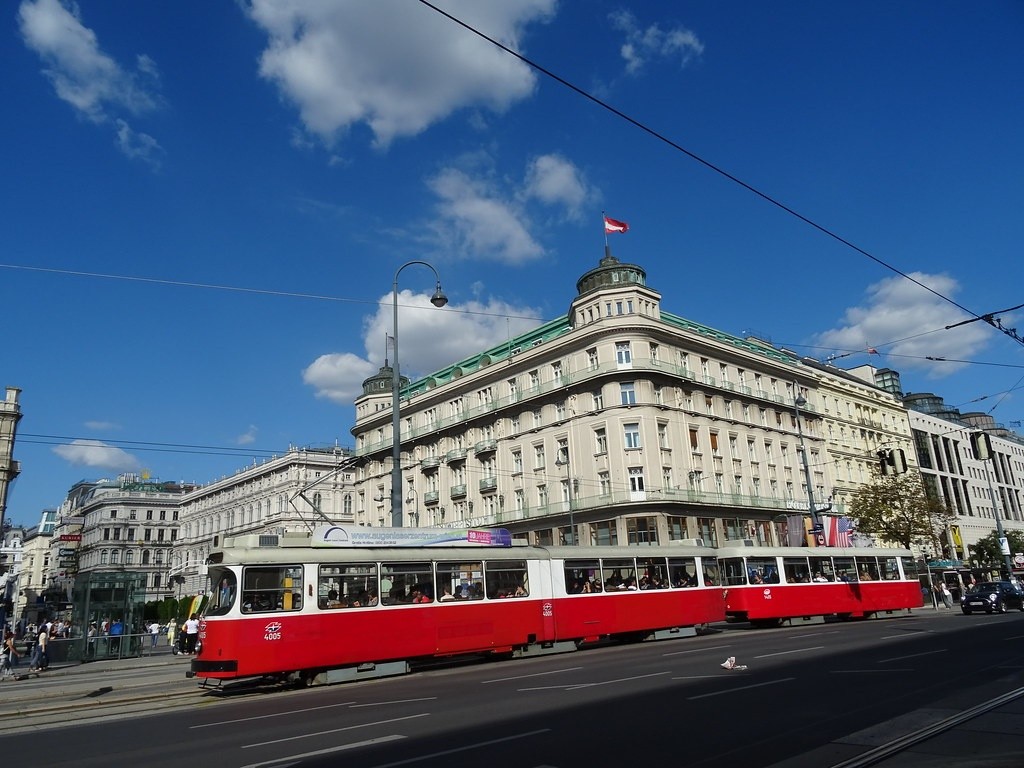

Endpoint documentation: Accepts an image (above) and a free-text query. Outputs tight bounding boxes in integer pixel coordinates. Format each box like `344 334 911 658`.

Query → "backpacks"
941 583 951 590
0 643 9 655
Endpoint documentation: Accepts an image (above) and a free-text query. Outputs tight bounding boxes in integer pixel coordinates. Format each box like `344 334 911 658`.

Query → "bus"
185 526 924 685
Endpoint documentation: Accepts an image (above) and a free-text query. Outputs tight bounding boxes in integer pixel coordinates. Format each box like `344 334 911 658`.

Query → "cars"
960 581 1024 615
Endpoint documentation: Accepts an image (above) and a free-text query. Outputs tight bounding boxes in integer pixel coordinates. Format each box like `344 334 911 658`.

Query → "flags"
785 512 854 548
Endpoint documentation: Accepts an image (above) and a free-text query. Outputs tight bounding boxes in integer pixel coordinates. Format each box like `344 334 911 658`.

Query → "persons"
0 613 202 682
607 564 874 586
326 570 604 608
928 578 1024 610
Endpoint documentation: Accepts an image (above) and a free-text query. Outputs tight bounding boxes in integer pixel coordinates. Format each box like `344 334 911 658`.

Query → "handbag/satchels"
968 583 975 589
40 652 48 667
165 628 169 632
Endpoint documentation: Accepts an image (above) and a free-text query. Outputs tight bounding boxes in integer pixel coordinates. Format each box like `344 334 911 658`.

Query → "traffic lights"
877 450 891 475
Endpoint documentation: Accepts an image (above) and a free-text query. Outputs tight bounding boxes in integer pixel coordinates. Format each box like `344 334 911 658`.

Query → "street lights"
393 261 448 526
792 379 829 548
556 448 575 546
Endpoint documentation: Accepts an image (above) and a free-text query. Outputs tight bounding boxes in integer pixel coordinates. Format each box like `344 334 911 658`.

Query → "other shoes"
178 651 183 655
184 652 189 656
35 668 40 672
28 667 33 671
192 651 195 655
